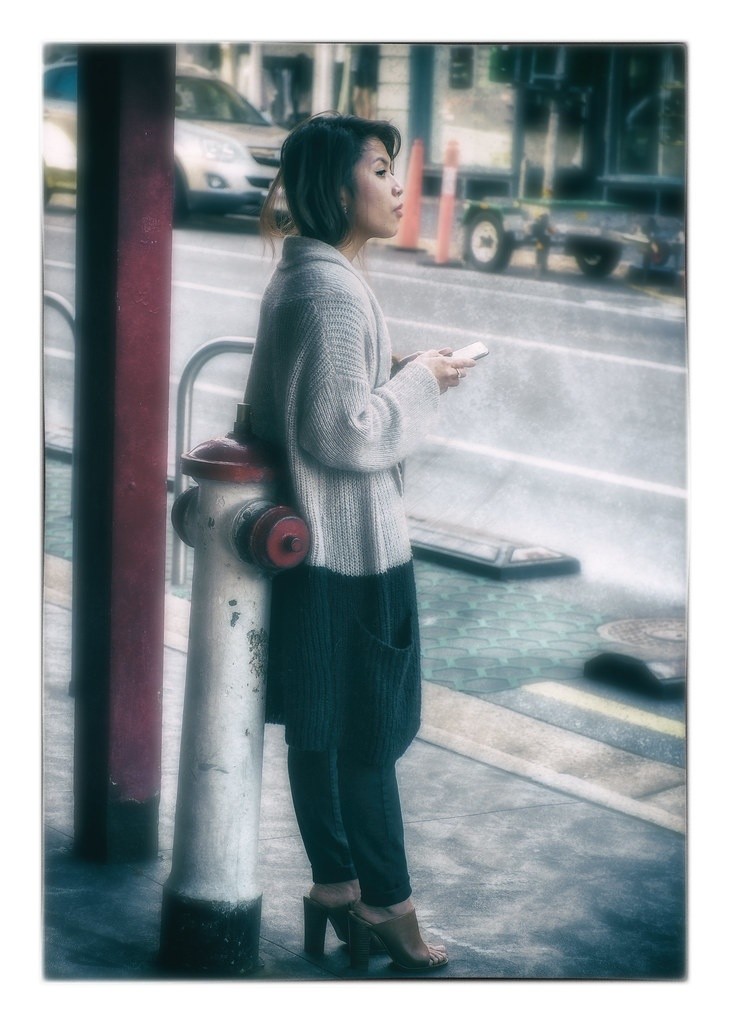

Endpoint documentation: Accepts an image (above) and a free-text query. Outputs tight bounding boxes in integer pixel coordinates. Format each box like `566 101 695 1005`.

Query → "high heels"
303 894 387 955
347 906 449 970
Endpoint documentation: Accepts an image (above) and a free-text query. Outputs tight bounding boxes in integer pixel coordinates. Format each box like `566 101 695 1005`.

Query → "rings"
455 368 461 380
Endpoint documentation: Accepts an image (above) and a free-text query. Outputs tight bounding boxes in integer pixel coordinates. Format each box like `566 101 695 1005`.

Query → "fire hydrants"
141 397 314 980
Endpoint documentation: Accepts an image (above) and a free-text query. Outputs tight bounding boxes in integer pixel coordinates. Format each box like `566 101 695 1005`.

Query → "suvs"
43 50 302 228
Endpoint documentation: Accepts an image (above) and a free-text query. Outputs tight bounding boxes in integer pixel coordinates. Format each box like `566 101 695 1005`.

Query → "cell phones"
444 339 489 361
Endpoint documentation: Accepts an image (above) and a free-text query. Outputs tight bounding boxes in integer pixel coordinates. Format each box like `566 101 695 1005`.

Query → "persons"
243 113 475 978
